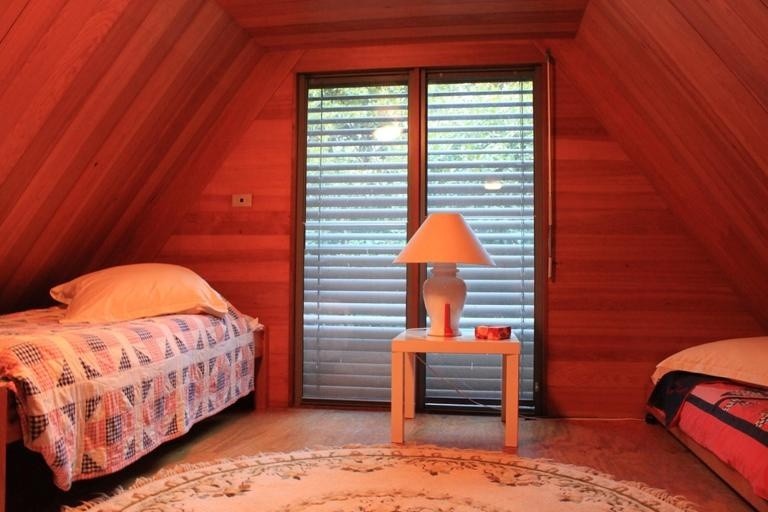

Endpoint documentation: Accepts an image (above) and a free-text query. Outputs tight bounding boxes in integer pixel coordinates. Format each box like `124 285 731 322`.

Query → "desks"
389 325 522 450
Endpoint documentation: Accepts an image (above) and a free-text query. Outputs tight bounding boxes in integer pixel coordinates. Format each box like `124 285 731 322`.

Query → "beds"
643 367 768 512
0 301 272 512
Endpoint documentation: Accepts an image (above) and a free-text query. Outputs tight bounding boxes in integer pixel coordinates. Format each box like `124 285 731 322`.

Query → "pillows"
48 261 234 326
647 334 767 387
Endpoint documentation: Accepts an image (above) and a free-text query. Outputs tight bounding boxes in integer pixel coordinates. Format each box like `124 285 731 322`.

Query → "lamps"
386 210 499 338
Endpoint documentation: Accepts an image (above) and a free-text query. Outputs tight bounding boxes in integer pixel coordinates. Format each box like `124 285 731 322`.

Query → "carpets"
50 435 707 511
